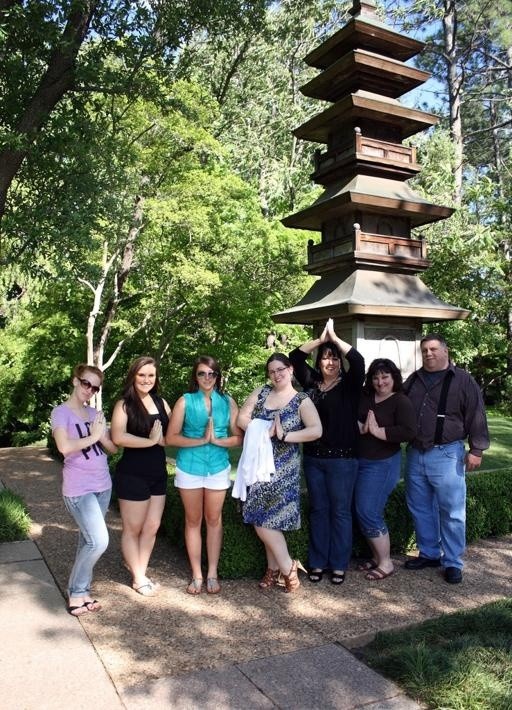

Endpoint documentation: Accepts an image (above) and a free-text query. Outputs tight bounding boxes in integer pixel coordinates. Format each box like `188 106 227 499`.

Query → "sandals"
309 559 395 585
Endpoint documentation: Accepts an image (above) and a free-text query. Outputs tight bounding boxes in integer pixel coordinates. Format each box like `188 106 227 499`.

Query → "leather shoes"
445 567 462 584
404 557 442 570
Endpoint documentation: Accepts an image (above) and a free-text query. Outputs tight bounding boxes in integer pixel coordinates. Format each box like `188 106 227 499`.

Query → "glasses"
268 367 287 375
78 377 100 394
196 370 217 377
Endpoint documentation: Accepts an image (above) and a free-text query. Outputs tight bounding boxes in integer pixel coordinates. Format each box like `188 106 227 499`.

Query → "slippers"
68 604 88 616
187 577 204 595
206 578 221 594
85 599 99 612
133 582 159 597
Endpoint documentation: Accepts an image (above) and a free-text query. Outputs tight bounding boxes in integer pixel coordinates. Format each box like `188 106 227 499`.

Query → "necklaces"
316 378 339 398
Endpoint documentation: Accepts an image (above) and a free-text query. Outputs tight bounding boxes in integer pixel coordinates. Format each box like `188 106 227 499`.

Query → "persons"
237 352 321 594
166 356 244 594
356 358 414 581
50 364 118 617
398 333 489 583
288 317 364 585
112 356 172 598
267 331 276 348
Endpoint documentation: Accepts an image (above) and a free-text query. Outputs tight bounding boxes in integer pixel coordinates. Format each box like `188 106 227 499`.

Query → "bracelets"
282 432 287 442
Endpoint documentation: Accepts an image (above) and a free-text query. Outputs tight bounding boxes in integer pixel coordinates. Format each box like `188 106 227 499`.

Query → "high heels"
258 567 280 590
282 559 308 594
